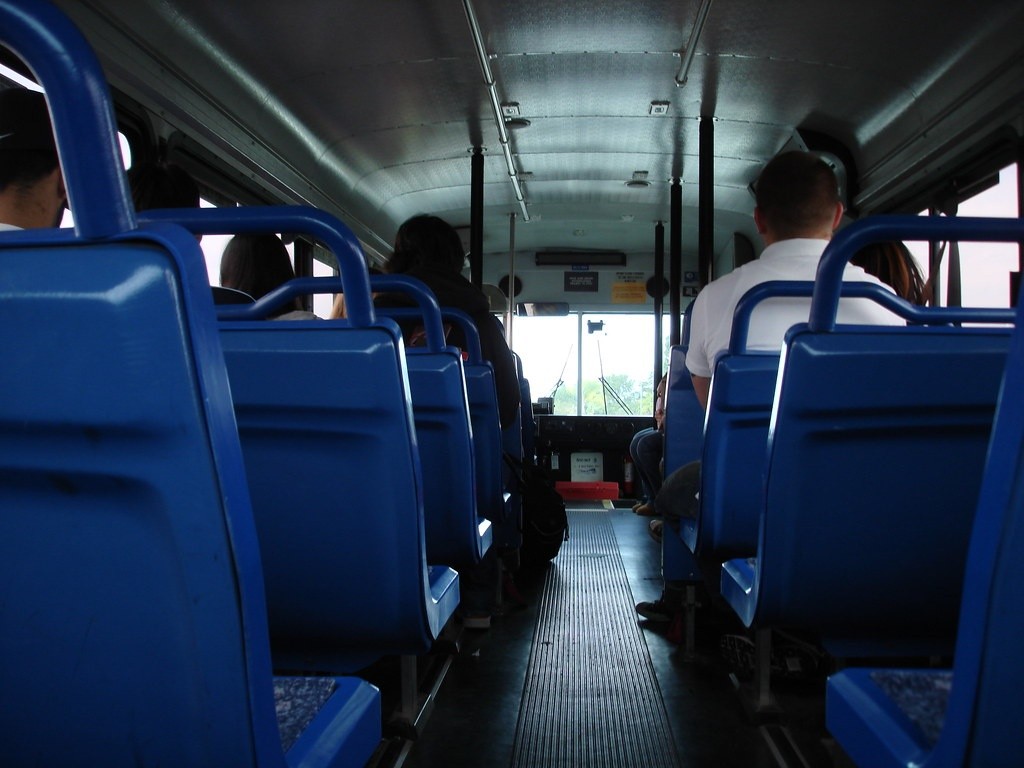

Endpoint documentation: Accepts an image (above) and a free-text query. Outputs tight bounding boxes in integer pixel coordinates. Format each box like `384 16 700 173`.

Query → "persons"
331 214 521 629
630 373 667 515
635 150 928 672
0 85 66 232
126 165 323 321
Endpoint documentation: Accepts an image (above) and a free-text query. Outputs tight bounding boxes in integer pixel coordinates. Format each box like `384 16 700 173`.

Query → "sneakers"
636 586 713 621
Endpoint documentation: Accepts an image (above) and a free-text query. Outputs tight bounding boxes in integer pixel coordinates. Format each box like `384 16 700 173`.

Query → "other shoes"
632 500 645 512
635 500 658 515
718 630 830 681
460 581 492 628
649 519 681 542
501 575 527 608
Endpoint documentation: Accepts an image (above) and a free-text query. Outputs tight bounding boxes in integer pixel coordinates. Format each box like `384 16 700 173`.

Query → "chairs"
664 217 1024 768
0 0 538 768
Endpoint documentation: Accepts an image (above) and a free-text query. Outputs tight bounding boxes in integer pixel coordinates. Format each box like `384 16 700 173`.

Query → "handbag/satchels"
521 485 568 556
